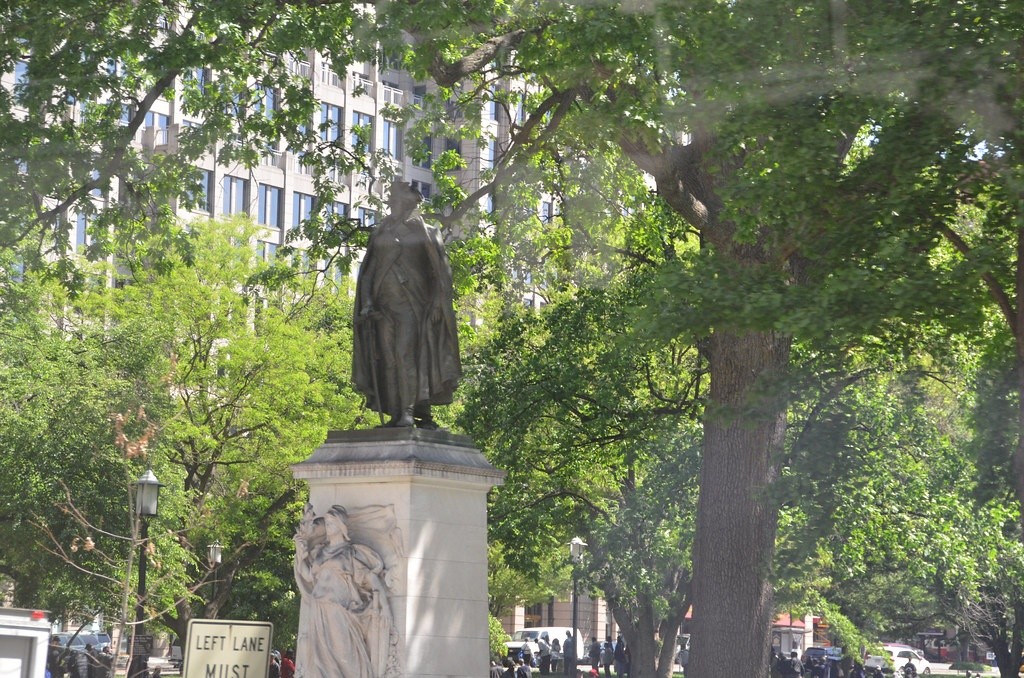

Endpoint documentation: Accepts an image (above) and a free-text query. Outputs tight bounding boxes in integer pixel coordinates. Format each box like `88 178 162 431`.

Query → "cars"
674 634 691 664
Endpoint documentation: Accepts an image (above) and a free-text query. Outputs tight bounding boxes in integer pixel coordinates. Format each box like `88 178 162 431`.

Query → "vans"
503 627 585 663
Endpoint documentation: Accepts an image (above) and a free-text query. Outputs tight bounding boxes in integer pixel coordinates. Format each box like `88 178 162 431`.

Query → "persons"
563 631 573 675
269 648 295 678
873 665 896 678
294 504 403 678
534 635 561 674
46 636 79 678
85 644 113 678
491 658 532 678
589 636 630 678
770 648 867 678
521 639 534 666
351 180 462 428
905 657 917 678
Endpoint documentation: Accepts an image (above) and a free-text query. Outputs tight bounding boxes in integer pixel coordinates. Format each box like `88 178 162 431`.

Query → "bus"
915 633 996 664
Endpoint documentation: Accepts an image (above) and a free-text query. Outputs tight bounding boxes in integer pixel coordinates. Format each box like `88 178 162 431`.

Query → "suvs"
801 647 844 678
862 642 932 678
51 632 113 654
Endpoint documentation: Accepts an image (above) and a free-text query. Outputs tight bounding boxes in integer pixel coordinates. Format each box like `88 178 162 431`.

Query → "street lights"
568 536 589 678
206 538 224 602
129 463 167 678
